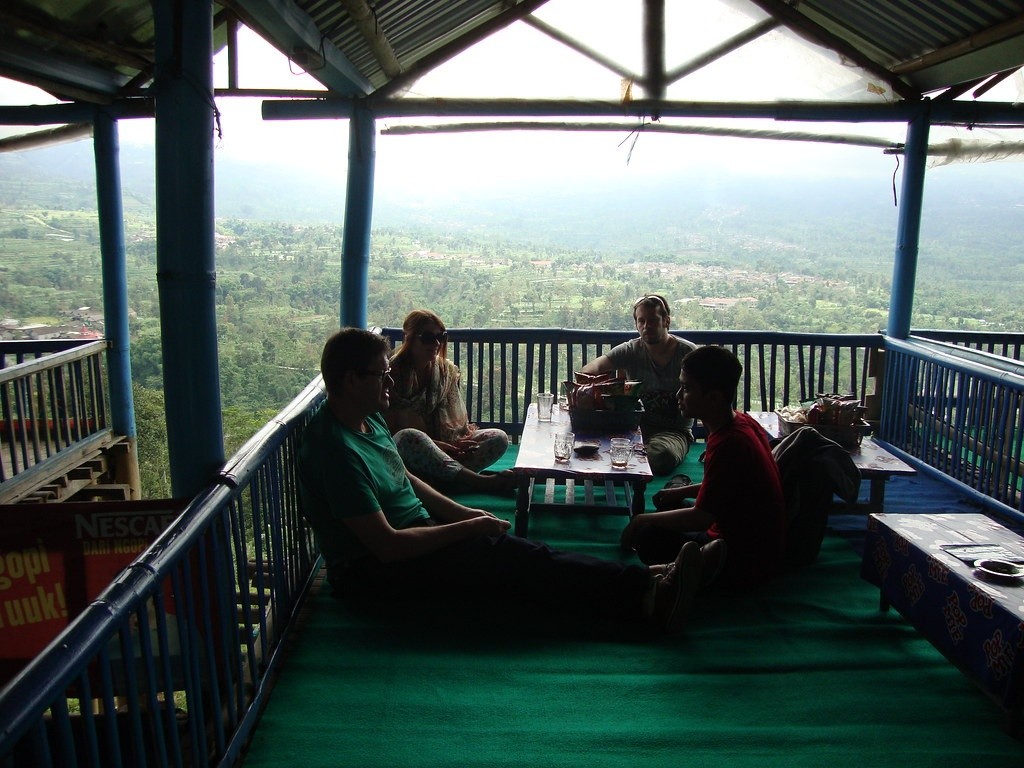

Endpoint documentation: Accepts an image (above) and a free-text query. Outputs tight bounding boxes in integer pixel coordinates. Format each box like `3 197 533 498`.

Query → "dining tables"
509 403 654 537
746 410 917 537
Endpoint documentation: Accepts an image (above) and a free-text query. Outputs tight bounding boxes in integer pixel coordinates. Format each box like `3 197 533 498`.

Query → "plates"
972 558 1024 580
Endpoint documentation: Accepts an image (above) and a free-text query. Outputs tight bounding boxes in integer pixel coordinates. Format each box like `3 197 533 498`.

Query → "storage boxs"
775 408 871 450
568 400 645 438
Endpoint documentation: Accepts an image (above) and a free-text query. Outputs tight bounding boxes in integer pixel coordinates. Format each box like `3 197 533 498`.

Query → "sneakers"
650 541 707 636
649 538 727 616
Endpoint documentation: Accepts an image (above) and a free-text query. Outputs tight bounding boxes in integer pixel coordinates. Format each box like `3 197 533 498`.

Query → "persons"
622 346 785 587
382 309 518 490
582 295 697 473
298 329 726 633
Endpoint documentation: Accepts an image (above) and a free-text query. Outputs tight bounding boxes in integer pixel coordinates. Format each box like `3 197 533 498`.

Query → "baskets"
774 406 871 447
569 398 645 431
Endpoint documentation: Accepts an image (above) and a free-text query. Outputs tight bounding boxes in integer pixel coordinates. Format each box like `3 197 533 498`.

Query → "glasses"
414 330 450 345
353 367 392 383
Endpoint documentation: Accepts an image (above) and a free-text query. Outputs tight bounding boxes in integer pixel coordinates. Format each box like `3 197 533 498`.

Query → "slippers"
436 478 473 495
654 473 694 512
478 469 523 492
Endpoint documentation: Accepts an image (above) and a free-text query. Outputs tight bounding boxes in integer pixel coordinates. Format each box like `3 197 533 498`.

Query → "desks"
860 512 1024 721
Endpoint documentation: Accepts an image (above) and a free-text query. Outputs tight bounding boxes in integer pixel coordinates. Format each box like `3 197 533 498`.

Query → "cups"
537 392 555 422
554 431 575 462
609 438 631 469
